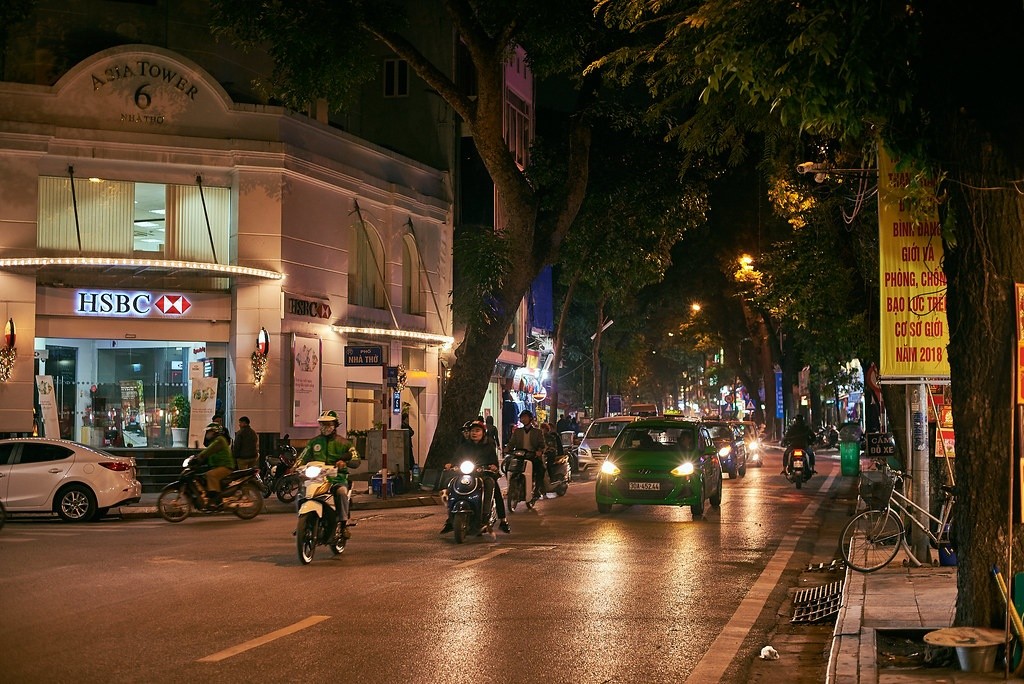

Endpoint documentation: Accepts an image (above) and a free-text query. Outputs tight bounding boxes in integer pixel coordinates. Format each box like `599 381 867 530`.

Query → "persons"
486 415 501 453
234 417 259 500
558 413 576 434
478 416 484 423
203 415 231 447
503 410 545 497
719 428 729 437
539 423 565 483
439 421 510 535
637 434 661 446
402 413 416 470
90 386 103 411
502 391 517 446
780 414 818 475
189 422 234 504
285 410 361 539
678 432 693 448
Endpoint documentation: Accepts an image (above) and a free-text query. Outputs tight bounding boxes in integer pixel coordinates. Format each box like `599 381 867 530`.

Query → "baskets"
861 471 895 510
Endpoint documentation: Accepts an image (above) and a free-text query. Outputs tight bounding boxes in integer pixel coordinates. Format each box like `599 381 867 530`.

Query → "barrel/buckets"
957 646 997 672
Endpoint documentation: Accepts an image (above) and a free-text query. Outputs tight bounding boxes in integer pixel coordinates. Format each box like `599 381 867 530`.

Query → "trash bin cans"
839 441 860 476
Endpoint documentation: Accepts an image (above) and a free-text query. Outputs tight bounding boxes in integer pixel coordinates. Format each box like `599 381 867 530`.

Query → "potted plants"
169 394 189 449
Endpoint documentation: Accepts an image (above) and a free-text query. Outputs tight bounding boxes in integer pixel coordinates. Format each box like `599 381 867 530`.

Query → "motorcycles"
812 425 841 450
281 460 358 565
258 438 301 502
158 455 266 524
779 438 817 489
501 447 543 512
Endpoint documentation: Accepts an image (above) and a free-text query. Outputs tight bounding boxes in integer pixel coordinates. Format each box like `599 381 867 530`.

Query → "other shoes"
338 526 352 538
206 494 223 505
779 470 786 475
812 470 818 475
498 521 512 534
438 523 456 534
533 491 541 497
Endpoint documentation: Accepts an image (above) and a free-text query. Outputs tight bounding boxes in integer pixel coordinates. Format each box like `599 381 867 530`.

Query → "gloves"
188 455 199 466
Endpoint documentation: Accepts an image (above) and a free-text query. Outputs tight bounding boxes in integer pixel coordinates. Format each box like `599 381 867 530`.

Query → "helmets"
203 423 223 432
317 409 341 425
519 410 536 419
459 420 489 430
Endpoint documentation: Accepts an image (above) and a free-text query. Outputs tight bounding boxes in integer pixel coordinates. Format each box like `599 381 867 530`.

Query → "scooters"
442 461 500 545
540 450 571 496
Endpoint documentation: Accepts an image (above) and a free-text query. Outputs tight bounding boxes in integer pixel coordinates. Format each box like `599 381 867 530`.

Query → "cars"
0 436 143 524
575 405 762 480
597 419 725 516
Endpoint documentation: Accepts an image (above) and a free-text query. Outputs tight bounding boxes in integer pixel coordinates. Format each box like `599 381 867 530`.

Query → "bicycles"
838 470 957 573
